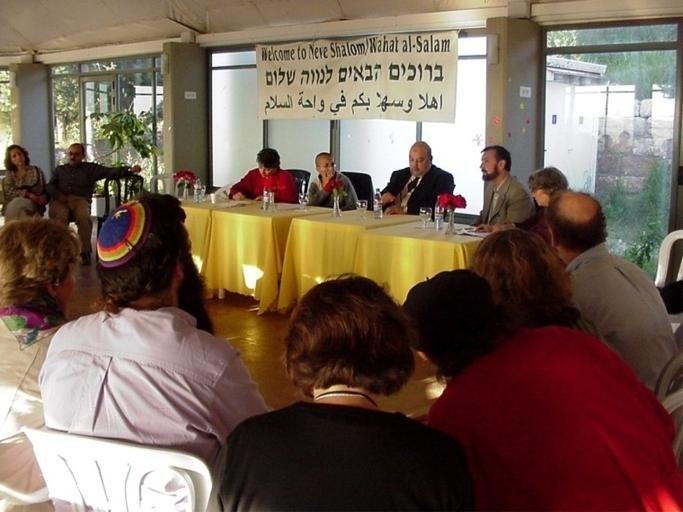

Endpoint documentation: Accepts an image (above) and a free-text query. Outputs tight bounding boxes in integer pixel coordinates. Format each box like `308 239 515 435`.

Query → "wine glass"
419 208 432 233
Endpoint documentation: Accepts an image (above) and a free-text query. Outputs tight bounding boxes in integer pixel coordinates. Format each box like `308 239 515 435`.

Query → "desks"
358 220 485 306
277 208 427 311
205 202 335 300
179 194 256 300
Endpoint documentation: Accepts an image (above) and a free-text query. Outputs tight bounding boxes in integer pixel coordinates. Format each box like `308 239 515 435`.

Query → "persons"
394 268 682 511
227 147 299 202
0 142 142 262
309 152 361 211
39 193 271 465
206 272 478 511
380 140 455 223
1 218 84 512
470 145 677 395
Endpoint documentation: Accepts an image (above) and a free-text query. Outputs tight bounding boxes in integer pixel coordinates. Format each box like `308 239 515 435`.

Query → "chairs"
653 229 683 465
20 424 215 512
96 172 144 262
655 355 683 467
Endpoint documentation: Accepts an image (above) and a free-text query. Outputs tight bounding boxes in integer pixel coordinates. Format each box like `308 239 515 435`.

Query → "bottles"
372 187 382 219
192 179 202 204
434 196 444 226
298 176 310 212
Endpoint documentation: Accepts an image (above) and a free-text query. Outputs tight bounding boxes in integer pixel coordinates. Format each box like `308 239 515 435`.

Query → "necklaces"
315 390 378 407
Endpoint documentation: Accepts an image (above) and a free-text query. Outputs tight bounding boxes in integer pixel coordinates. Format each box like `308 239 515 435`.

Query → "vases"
437 195 467 234
182 183 189 200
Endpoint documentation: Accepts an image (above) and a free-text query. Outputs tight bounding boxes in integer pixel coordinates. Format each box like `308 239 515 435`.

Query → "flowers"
173 169 196 199
324 178 349 216
261 178 277 209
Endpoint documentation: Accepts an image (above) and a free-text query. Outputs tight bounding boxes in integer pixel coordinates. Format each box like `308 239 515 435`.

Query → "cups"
210 194 216 204
263 187 275 212
354 199 368 222
